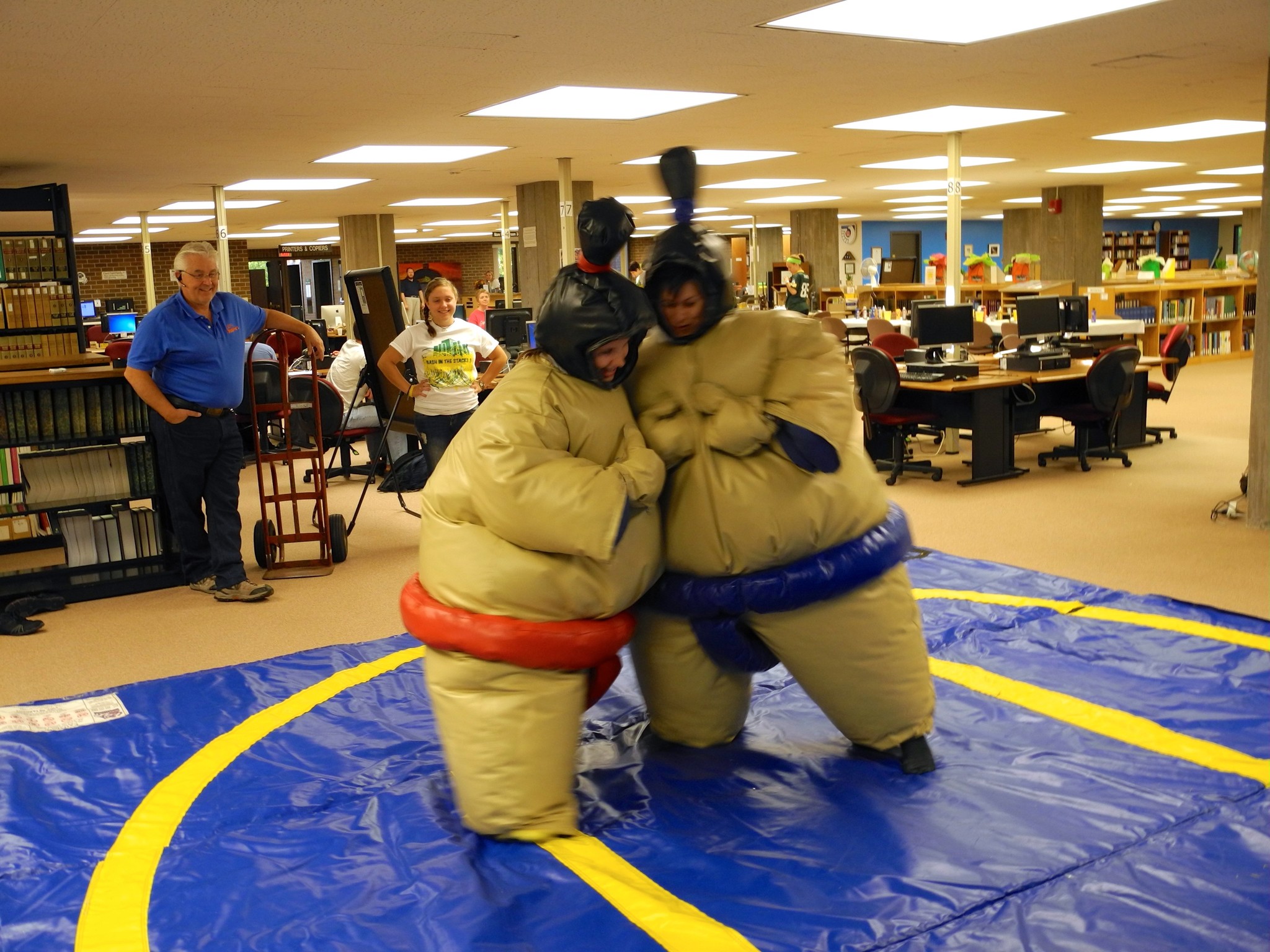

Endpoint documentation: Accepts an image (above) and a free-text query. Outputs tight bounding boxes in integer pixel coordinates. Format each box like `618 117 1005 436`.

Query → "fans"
861 257 880 288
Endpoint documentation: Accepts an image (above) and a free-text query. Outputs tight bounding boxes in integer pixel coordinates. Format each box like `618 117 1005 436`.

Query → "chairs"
85 325 116 348
961 322 1021 359
1037 322 1192 471
815 311 944 487
238 359 380 484
105 341 132 368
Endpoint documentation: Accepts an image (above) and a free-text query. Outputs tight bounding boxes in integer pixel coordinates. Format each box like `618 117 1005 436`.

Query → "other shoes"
4 593 65 616
260 438 274 451
0 612 45 635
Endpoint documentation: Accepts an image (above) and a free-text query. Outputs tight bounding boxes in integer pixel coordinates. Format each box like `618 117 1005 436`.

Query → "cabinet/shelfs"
0 181 86 354
818 279 1075 319
0 365 190 615
1078 229 1258 364
771 262 809 298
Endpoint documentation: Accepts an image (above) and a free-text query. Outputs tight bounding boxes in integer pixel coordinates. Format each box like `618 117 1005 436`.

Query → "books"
1102 237 1156 271
1172 235 1189 268
0 445 161 567
965 296 1000 314
1163 293 1257 357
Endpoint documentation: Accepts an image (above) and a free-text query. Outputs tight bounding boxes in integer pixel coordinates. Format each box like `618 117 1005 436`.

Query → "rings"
419 387 420 389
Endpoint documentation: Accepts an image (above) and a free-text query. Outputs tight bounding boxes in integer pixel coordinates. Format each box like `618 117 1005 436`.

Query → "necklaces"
432 318 454 332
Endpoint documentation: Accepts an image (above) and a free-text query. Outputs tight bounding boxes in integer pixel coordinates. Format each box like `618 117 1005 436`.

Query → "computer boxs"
998 345 1094 373
904 349 979 379
479 360 516 374
293 355 337 371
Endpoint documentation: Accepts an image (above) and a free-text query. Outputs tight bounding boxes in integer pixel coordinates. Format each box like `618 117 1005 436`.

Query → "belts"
167 396 232 420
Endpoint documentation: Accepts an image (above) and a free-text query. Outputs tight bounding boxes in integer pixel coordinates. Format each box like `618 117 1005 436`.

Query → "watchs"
479 380 485 390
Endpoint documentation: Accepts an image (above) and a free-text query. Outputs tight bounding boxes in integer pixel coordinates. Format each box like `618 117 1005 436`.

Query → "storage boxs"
1160 257 1176 280
1110 259 1126 280
771 286 787 306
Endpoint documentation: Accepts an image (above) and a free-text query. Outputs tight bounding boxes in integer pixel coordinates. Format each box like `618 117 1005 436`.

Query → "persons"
377 278 507 472
400 267 425 326
617 147 937 775
468 271 501 329
244 323 407 470
400 195 663 843
629 262 646 287
124 243 324 601
784 254 809 316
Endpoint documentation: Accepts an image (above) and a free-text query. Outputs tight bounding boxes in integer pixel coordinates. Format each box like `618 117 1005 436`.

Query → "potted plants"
845 273 853 287
1215 257 1227 276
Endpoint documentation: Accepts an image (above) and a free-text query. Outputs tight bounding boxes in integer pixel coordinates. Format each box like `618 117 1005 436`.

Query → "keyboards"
900 372 945 382
1013 347 1070 356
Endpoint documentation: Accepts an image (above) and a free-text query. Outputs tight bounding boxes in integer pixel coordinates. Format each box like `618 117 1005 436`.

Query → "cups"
1013 309 1017 321
975 311 984 322
884 311 891 321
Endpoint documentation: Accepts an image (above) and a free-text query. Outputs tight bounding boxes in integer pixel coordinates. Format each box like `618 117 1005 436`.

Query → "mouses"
953 375 968 382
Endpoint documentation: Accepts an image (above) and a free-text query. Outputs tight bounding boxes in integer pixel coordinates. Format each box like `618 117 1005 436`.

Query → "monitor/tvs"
79 298 138 339
1016 295 1088 347
486 307 537 363
910 298 974 364
305 305 346 355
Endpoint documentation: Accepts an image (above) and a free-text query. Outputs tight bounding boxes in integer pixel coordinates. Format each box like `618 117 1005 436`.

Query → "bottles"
855 307 860 319
862 306 867 319
998 306 1003 320
868 304 886 320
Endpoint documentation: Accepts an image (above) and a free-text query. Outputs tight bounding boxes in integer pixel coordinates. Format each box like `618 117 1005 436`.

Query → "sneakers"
214 578 274 602
190 574 218 594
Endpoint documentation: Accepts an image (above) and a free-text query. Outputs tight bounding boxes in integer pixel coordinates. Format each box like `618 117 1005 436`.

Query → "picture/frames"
964 243 1000 258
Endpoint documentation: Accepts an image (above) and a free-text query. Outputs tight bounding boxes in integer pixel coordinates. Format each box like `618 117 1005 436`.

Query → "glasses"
179 270 219 279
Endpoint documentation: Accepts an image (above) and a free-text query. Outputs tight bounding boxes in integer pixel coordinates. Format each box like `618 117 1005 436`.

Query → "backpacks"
377 449 428 493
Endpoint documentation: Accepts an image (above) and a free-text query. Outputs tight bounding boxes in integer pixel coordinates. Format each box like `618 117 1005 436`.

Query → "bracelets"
407 385 414 397
786 281 789 283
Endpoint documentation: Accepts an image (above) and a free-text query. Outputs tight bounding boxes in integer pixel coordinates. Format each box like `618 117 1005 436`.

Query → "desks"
327 333 346 352
841 319 904 352
268 369 330 443
863 356 1182 487
987 319 1146 351
83 315 102 325
85 347 105 354
